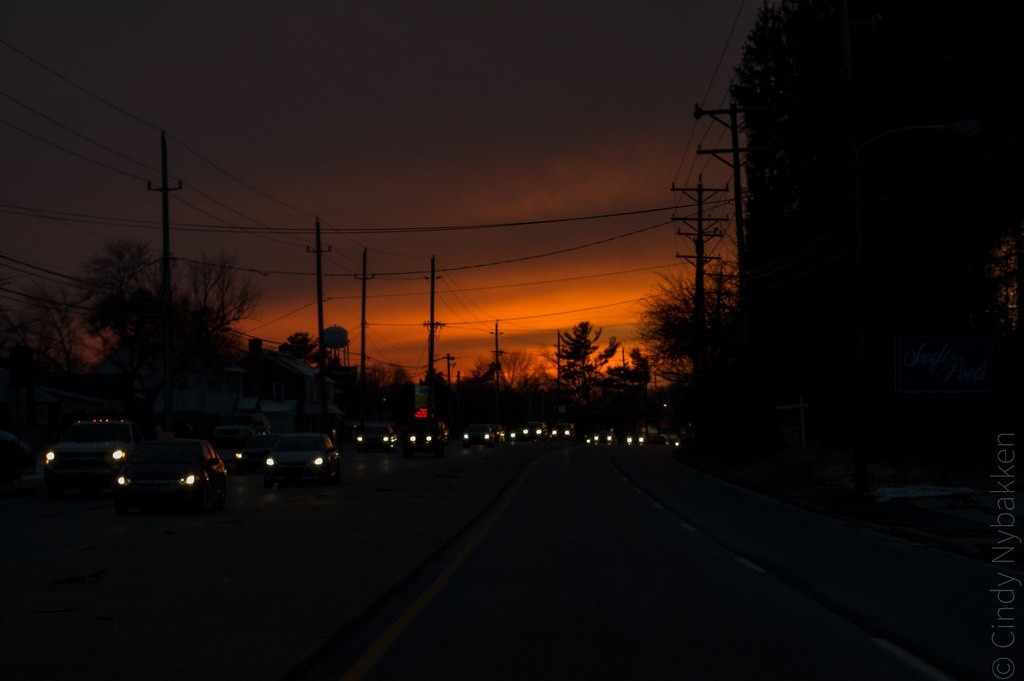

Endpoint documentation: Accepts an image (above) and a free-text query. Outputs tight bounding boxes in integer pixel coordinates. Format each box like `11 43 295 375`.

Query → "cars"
263 433 341 487
0 429 33 484
114 439 226 515
524 422 549 442
357 424 398 453
462 424 506 448
403 424 445 460
245 433 285 465
550 423 575 440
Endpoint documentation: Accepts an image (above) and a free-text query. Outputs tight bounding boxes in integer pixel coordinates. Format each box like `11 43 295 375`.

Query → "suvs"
44 419 140 488
